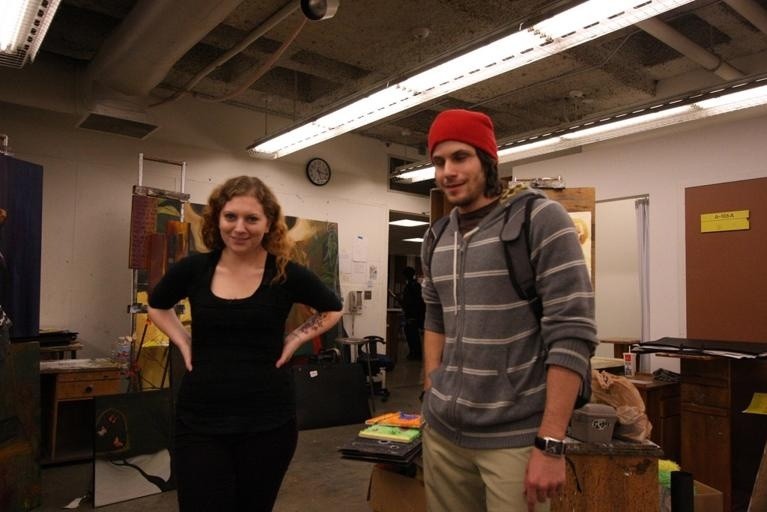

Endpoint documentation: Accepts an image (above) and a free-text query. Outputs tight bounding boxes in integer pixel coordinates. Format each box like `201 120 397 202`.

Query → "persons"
400 266 425 362
147 175 344 512
419 109 601 512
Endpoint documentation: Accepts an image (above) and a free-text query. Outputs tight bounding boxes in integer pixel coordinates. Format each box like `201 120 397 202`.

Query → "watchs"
534 434 568 459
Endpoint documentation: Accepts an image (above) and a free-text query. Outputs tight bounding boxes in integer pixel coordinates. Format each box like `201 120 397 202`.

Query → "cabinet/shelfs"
611 349 767 512
40 357 133 466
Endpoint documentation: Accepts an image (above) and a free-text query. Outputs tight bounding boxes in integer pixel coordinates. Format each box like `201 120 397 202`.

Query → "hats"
427 108 498 164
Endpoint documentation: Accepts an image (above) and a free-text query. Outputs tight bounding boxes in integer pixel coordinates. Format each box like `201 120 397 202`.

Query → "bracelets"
419 389 427 402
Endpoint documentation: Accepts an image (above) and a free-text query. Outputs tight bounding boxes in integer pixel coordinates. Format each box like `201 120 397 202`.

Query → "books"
335 410 426 460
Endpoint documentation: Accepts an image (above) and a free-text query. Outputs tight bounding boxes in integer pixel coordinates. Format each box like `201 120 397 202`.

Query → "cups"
624 352 636 378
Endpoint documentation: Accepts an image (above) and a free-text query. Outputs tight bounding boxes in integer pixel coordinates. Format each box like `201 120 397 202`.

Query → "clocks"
306 157 331 186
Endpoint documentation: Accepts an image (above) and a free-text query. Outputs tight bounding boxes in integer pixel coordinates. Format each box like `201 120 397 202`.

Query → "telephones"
349 290 363 314
